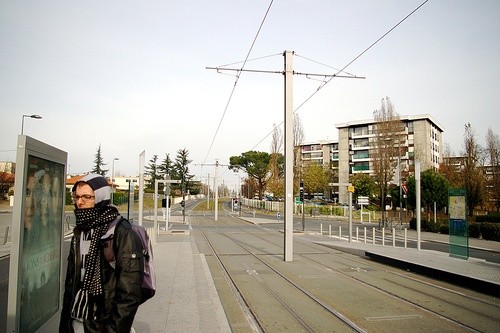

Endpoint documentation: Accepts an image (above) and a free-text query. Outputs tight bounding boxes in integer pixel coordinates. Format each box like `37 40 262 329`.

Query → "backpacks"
99 215 156 304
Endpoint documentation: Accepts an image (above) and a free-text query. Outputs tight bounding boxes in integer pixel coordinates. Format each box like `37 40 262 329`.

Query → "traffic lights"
299 181 304 202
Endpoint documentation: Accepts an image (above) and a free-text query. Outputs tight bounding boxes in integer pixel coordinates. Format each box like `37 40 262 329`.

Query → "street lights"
111 157 120 204
20 113 42 136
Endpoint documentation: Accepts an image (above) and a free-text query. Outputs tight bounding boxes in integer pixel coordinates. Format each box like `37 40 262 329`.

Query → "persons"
233 197 236 207
58 173 145 333
181 199 185 216
19 158 63 333
234 200 239 212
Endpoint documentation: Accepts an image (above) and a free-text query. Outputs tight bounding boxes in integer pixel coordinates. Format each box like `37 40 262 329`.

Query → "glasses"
73 193 95 202
